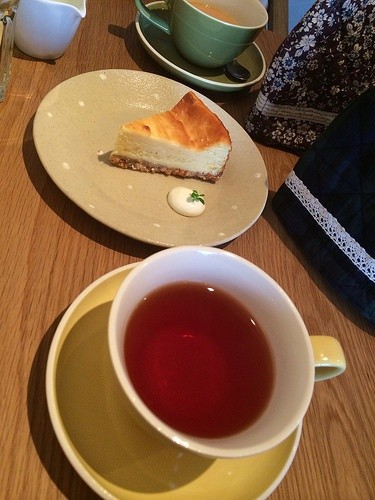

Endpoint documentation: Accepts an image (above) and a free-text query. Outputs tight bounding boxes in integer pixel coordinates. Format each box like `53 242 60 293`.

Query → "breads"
108 90 232 182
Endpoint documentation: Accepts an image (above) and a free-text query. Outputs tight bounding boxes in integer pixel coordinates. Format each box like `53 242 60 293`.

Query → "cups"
11 0 87 61
108 245 347 460
135 0 269 70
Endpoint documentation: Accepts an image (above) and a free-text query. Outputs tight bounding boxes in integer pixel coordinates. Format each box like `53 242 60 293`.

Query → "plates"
135 0 266 91
31 69 270 247
45 260 310 500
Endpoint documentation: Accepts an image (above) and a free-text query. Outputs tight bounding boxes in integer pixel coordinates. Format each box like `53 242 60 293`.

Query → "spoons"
226 60 250 82
